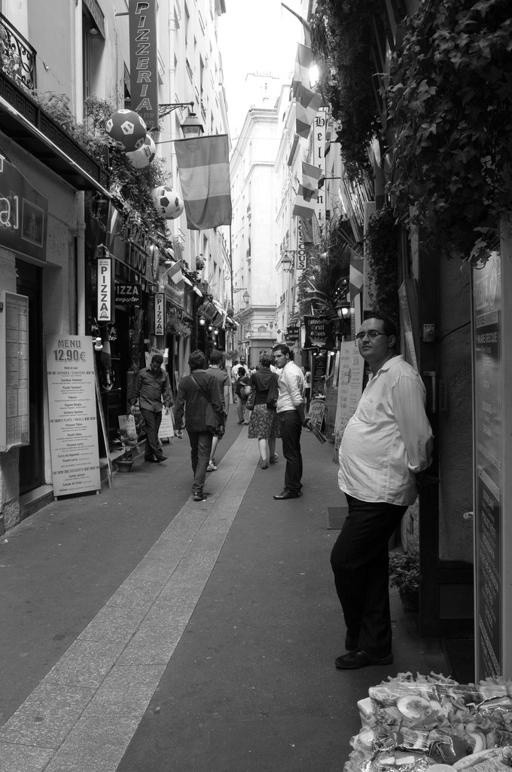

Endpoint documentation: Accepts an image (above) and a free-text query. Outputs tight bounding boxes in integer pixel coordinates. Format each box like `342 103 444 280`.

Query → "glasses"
355 330 385 338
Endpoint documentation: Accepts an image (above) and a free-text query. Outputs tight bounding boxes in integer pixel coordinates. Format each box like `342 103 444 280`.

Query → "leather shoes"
273 493 299 499
193 492 202 500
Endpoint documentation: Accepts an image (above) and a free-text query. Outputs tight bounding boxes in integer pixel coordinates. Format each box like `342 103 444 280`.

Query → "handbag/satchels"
267 383 277 407
205 402 225 439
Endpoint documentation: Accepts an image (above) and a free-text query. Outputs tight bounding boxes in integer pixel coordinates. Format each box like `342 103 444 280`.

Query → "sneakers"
146 454 167 463
336 650 393 669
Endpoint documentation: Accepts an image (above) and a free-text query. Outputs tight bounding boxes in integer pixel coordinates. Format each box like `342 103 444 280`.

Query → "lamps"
198 315 221 338
335 300 356 318
91 336 104 352
157 101 213 152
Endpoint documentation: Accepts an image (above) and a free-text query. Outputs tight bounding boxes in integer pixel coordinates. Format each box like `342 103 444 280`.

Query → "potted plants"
112 428 139 472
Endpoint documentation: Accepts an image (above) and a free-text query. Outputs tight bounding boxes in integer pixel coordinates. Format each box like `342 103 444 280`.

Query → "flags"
174 135 232 230
292 42 327 204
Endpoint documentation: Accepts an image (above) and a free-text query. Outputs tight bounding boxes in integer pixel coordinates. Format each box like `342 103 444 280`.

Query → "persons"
128 342 312 502
328 310 436 671
